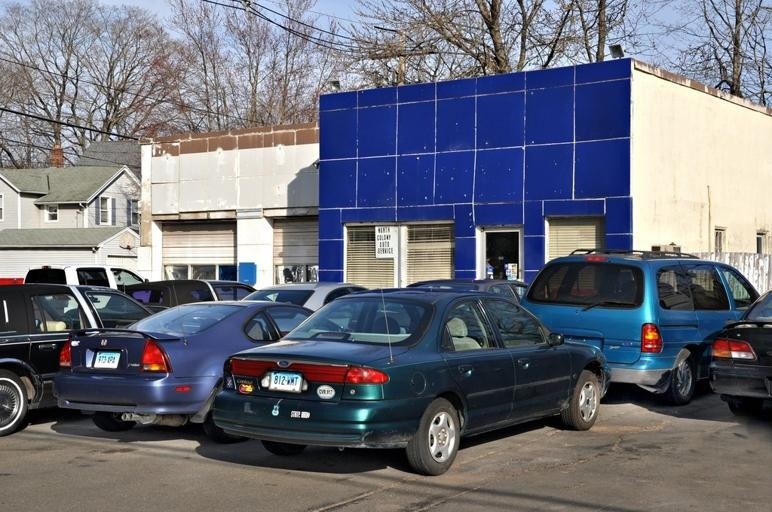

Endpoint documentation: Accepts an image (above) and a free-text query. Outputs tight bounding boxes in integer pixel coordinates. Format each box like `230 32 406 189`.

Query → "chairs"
441 317 482 352
39 320 66 332
371 316 400 334
199 319 217 330
622 280 674 308
244 319 267 341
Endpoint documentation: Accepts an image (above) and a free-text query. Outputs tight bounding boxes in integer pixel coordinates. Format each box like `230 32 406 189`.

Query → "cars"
54 296 347 445
213 287 610 476
704 290 772 416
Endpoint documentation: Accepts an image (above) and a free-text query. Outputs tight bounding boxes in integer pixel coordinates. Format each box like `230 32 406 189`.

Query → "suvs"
0 283 156 437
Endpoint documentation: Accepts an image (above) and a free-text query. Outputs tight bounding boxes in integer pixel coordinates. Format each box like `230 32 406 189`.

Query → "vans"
23 264 149 314
521 247 760 406
